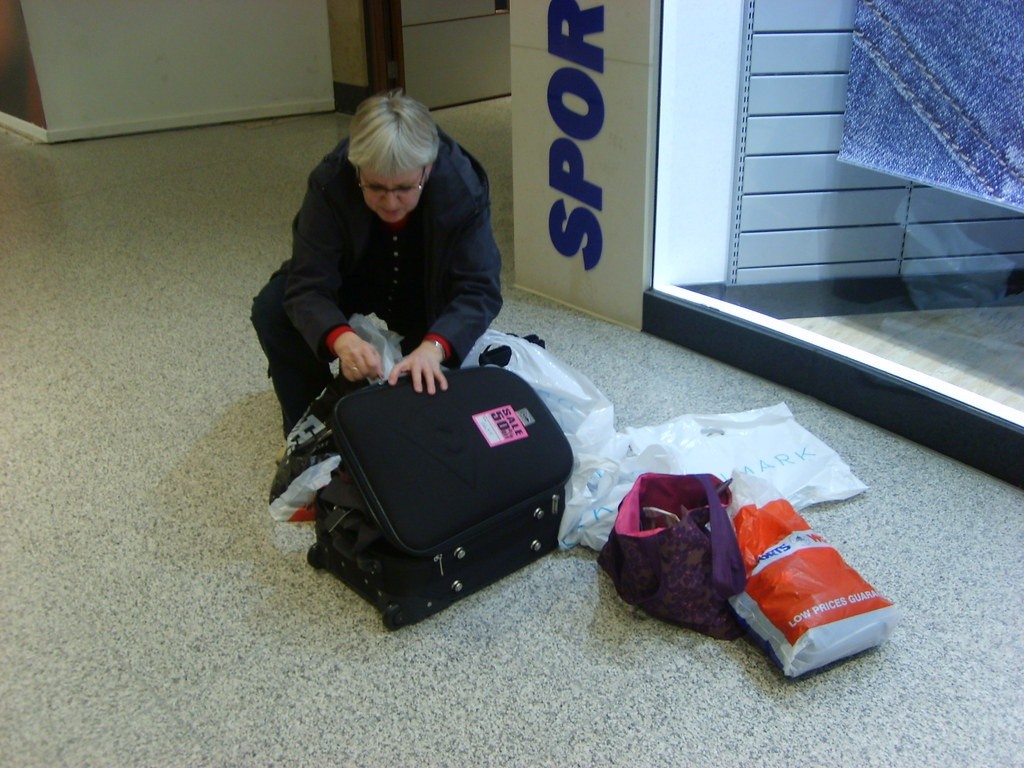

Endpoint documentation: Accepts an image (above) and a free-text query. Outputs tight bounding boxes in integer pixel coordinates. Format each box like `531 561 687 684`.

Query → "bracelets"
422 338 445 359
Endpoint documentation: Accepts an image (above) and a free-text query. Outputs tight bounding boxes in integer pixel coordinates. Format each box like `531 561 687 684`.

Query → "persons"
250 90 504 465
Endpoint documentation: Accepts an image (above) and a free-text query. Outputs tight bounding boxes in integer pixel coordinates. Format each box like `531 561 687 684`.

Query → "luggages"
306 364 574 632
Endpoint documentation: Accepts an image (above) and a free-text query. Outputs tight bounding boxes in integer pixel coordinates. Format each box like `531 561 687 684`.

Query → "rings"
350 367 358 371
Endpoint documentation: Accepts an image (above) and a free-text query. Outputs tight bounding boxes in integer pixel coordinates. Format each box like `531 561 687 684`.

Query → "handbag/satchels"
704 468 898 678
268 312 406 522
460 331 868 551
596 472 749 641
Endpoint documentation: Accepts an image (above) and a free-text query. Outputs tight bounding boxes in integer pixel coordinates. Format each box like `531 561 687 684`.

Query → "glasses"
358 165 425 194
